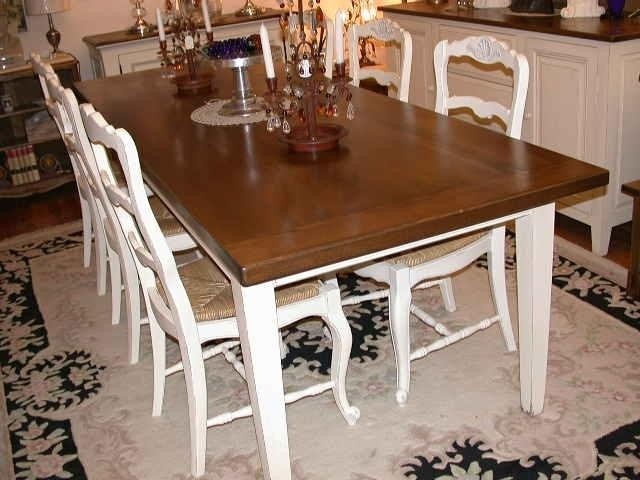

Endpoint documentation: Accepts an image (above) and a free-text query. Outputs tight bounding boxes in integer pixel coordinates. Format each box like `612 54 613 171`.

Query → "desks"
74 55 609 476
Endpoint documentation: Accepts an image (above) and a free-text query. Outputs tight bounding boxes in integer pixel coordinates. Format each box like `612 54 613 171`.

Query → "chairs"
79 101 360 477
31 53 155 297
339 35 530 406
350 18 413 102
46 72 203 364
283 15 333 78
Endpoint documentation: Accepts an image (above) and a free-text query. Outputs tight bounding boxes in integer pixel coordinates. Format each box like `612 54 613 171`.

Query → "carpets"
2 213 640 477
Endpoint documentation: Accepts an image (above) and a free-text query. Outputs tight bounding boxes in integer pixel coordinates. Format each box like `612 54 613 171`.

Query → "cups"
164 0 222 27
1 94 14 113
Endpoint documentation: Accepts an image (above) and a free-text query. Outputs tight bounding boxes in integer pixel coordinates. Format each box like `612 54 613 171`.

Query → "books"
4 144 41 186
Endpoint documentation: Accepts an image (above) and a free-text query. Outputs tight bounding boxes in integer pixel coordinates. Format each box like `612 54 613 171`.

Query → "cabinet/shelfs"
1 50 84 199
378 11 639 258
85 9 310 82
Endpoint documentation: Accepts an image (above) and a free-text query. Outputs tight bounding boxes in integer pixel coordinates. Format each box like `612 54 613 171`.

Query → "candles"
335 9 344 62
260 21 276 79
200 0 212 32
157 8 166 41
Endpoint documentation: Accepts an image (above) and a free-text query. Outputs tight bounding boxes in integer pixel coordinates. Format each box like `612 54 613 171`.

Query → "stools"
617 182 640 301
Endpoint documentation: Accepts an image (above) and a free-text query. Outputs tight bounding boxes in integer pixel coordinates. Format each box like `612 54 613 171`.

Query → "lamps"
25 0 71 57
